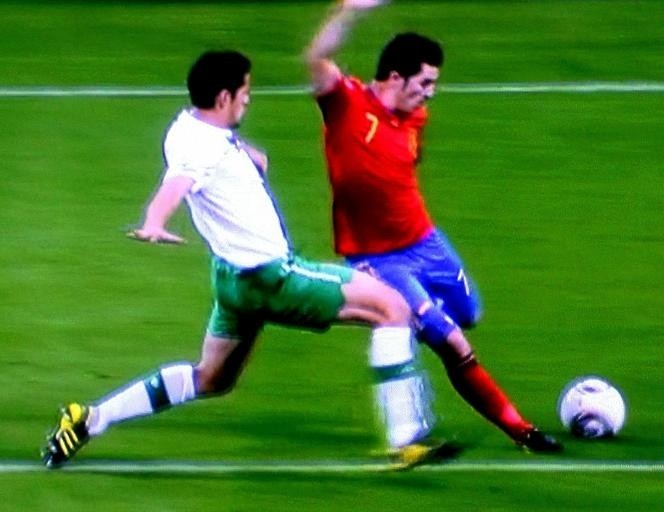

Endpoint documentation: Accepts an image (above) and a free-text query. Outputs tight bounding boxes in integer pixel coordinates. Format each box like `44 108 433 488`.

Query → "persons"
42 50 464 472
300 0 567 454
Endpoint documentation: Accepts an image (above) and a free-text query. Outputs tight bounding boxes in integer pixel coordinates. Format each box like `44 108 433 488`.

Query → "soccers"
556 375 627 439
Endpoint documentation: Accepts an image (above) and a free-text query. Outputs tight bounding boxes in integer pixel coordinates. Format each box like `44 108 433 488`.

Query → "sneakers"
384 442 462 472
38 403 94 470
514 424 566 456
